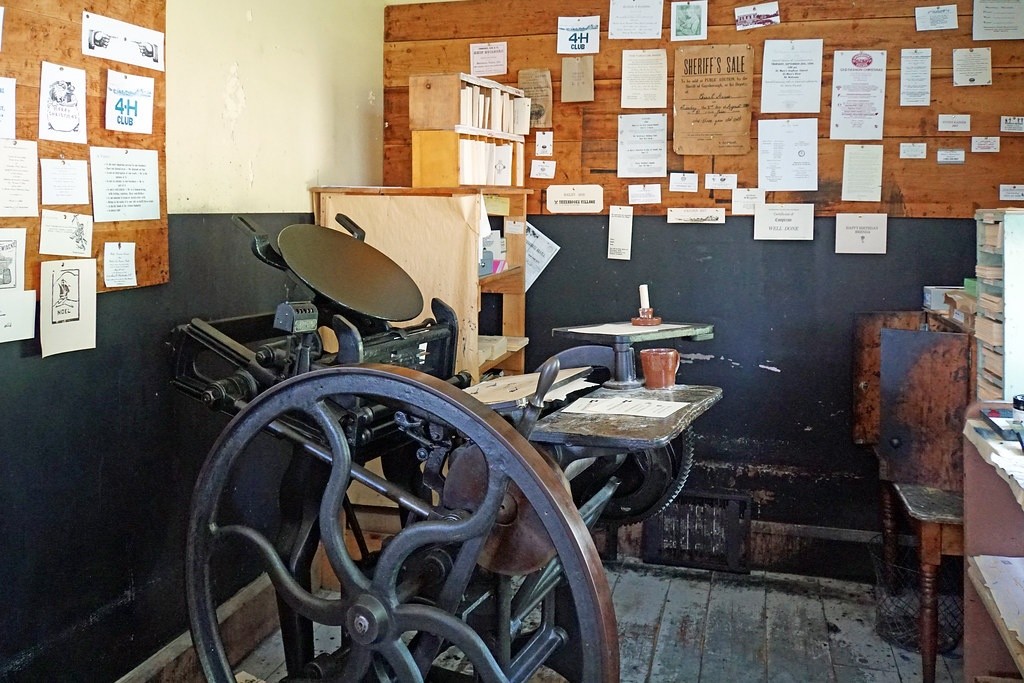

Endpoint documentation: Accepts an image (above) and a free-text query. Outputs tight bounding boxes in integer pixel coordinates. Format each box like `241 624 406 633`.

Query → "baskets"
867 535 965 655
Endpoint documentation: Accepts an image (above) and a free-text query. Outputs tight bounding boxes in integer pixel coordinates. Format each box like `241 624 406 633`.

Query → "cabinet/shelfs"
848 306 1024 683
307 70 534 385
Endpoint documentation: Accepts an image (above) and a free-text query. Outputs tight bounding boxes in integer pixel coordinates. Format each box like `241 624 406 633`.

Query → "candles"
639 284 649 309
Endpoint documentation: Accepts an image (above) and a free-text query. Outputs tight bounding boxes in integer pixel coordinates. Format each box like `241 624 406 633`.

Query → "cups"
641 348 680 389
1013 394 1024 426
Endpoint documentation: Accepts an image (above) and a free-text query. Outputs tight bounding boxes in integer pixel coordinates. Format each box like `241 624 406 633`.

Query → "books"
460 86 531 135
459 139 525 186
974 222 1004 403
